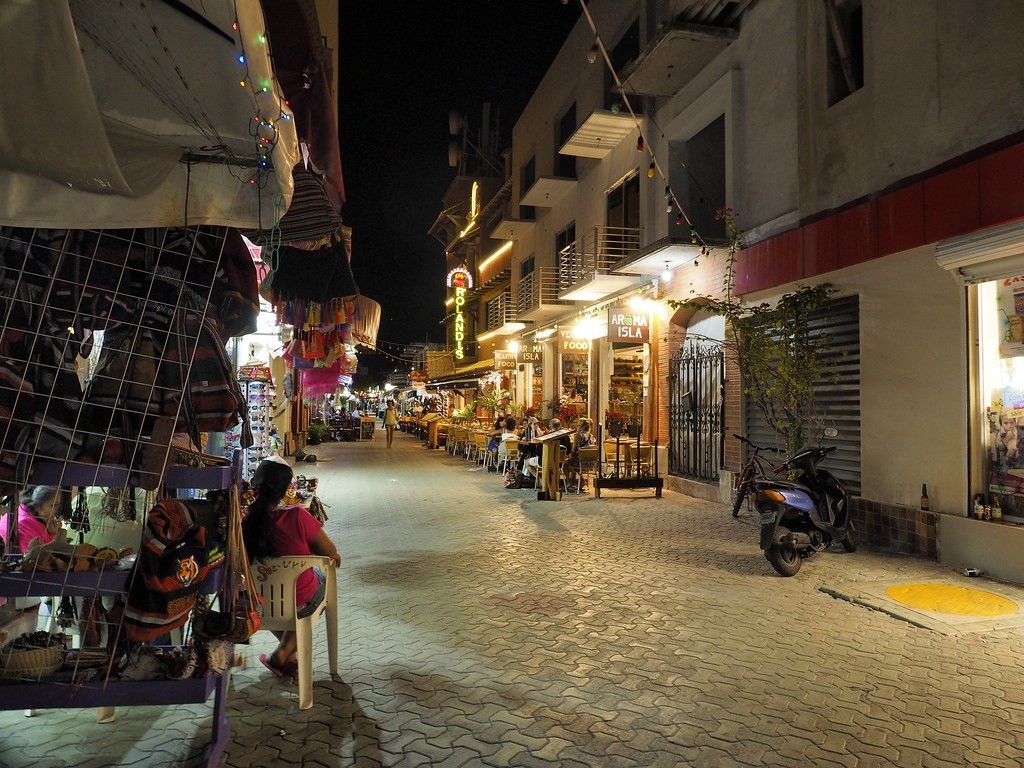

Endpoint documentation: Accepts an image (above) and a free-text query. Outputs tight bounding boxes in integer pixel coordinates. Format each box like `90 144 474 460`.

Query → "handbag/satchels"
193 590 267 642
504 467 522 489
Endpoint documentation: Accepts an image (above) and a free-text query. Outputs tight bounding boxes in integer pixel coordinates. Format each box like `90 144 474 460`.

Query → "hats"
528 417 540 424
253 459 292 498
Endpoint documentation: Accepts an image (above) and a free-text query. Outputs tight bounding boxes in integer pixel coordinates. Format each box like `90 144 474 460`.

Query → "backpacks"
85 311 165 471
151 291 253 467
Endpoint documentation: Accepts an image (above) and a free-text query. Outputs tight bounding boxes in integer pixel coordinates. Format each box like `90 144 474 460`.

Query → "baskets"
0 644 64 679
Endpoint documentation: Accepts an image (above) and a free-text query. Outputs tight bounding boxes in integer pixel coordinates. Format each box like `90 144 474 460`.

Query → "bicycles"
731 432 788 516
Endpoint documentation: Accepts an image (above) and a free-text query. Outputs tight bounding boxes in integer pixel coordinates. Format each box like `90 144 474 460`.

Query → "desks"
330 417 359 432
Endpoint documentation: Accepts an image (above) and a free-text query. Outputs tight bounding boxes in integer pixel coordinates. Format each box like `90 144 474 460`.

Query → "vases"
611 423 622 437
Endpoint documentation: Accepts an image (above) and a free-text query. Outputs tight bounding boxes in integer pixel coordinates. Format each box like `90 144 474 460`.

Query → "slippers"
259 653 285 678
282 655 297 675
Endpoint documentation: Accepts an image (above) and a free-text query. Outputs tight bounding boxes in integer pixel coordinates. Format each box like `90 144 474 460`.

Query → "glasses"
224 381 277 474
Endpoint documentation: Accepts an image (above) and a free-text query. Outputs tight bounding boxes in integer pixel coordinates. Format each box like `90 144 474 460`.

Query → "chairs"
243 555 338 710
446 425 654 494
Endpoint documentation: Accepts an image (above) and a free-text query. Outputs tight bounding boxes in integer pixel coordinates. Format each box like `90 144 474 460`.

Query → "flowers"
525 406 535 417
604 409 623 425
558 407 576 427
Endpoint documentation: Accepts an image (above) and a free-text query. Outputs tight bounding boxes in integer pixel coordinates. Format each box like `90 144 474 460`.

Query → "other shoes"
389 445 391 448
387 445 389 448
560 485 573 492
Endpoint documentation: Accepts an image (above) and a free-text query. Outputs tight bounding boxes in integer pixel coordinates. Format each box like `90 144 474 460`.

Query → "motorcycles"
740 427 858 579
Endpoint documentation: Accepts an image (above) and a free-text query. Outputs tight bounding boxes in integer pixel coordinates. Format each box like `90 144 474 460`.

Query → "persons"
62 486 148 648
0 486 61 605
325 406 366 418
489 417 596 489
382 400 398 448
241 460 341 677
413 403 423 412
449 404 458 416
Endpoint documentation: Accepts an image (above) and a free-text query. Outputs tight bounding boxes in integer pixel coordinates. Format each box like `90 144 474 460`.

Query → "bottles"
992 495 1002 522
921 484 929 510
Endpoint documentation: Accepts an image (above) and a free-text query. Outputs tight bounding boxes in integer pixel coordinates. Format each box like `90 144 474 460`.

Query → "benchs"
328 428 354 442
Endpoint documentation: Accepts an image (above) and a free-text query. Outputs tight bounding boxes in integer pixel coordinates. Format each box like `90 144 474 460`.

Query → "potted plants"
625 391 644 437
308 424 329 444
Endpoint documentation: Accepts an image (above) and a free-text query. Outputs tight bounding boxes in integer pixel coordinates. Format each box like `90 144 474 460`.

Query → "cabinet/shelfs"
561 361 589 395
611 361 643 393
0 449 244 768
